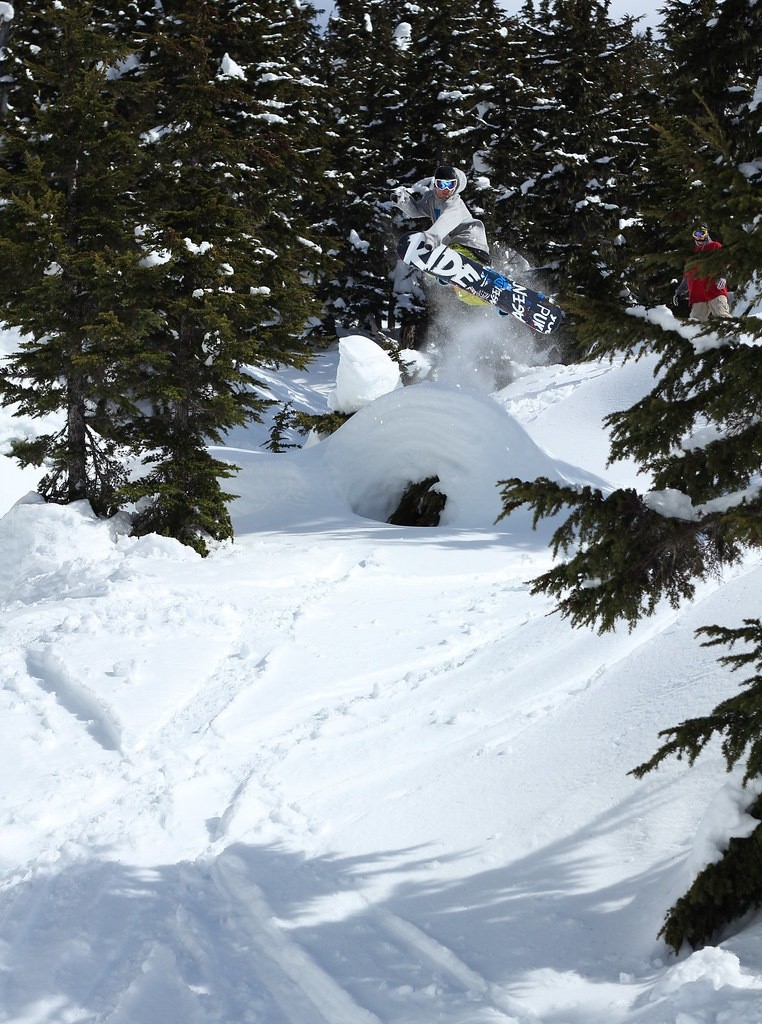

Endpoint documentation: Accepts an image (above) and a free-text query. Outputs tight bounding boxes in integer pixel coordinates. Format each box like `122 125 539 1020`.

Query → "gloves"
715 277 726 290
673 294 678 306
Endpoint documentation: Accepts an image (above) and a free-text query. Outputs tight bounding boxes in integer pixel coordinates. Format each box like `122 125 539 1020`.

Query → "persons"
390 166 509 316
674 221 733 326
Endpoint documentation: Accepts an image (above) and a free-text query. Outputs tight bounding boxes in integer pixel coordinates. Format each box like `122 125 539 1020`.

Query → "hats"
435 166 458 180
692 222 708 240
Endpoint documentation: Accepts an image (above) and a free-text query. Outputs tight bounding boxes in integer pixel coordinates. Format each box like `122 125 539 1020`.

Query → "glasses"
435 179 457 190
694 231 704 236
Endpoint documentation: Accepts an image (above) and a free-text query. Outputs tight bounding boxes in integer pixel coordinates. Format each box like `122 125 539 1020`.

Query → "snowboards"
397 230 563 336
